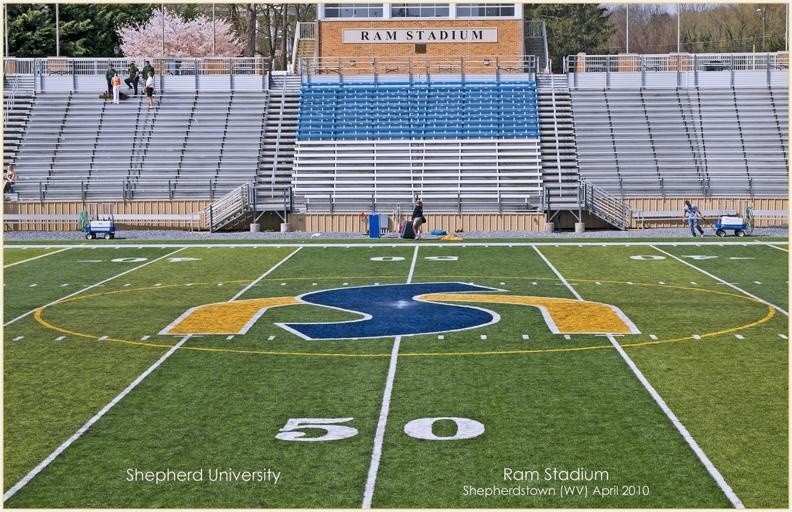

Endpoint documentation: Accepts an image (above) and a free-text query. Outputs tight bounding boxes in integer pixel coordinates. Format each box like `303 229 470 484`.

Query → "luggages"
402 221 416 239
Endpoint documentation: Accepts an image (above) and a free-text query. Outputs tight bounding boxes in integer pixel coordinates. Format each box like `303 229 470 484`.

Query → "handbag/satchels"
421 215 426 224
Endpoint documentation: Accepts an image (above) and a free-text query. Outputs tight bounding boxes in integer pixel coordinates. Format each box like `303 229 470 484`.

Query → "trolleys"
703 216 746 237
85 214 115 239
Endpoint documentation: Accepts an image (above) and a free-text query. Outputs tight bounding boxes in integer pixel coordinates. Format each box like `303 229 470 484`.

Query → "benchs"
3 82 789 213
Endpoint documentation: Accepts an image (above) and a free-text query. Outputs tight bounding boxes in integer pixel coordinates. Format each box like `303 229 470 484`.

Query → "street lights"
756 8 765 51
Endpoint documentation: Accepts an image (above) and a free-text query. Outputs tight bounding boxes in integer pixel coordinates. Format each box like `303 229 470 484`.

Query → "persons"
105 63 117 95
683 201 705 238
111 72 121 105
124 63 140 98
141 61 154 93
412 200 426 239
6 164 16 192
143 71 155 108
